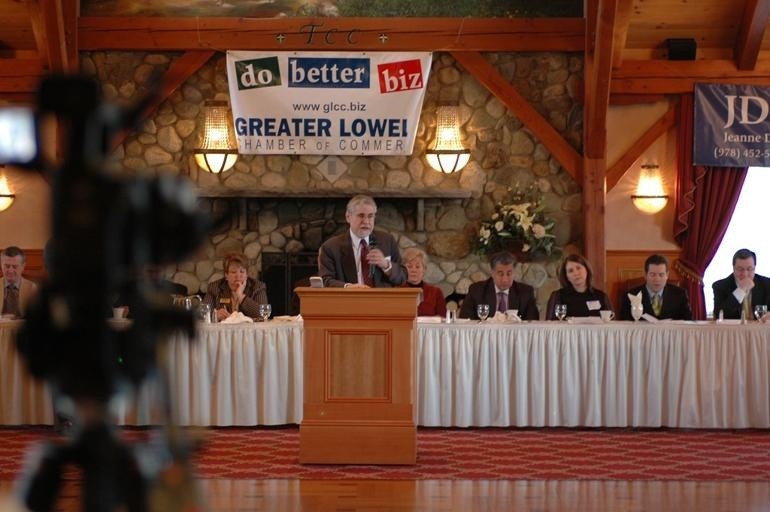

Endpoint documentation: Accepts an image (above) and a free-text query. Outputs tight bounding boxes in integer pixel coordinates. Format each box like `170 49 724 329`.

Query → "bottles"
212 308 218 322
741 311 746 324
718 310 724 325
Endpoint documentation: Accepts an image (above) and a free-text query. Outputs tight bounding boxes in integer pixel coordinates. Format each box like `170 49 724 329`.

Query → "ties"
744 291 752 321
498 293 506 314
652 292 661 316
360 239 373 288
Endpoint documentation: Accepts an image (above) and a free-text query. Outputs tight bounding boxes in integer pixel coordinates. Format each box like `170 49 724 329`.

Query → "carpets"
2 427 768 484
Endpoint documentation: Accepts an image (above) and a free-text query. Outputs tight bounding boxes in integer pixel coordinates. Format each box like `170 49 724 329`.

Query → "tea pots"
168 294 203 316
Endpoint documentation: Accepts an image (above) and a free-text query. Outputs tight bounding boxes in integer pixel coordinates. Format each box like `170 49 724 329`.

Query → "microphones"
368 234 376 278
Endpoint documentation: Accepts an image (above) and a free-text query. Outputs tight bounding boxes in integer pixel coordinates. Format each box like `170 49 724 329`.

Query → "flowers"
469 178 560 256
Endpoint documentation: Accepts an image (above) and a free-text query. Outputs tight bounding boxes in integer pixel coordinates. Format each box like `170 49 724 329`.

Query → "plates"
108 319 129 323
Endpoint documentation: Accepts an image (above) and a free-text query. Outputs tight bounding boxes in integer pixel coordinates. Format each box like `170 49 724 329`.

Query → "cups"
754 305 767 320
600 310 615 322
200 304 211 324
446 303 456 323
112 307 124 320
503 309 519 320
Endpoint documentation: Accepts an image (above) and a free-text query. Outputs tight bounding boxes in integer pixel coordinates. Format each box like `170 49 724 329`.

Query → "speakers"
667 38 697 60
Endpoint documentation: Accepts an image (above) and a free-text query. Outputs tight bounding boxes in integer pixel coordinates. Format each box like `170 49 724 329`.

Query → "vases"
504 238 529 263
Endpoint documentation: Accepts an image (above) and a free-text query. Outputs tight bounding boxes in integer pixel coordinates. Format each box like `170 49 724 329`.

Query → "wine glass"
555 304 567 324
631 304 643 324
477 305 490 324
258 304 271 319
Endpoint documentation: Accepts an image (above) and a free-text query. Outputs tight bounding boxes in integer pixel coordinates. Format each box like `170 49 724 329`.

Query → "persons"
618 254 693 320
200 251 269 324
546 254 615 320
404 247 447 317
317 195 408 289
459 251 539 320
0 246 40 317
711 248 770 319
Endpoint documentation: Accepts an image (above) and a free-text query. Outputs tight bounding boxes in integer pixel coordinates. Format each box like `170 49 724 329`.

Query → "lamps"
194 101 240 175
424 100 474 174
630 161 670 214
0 169 14 215
